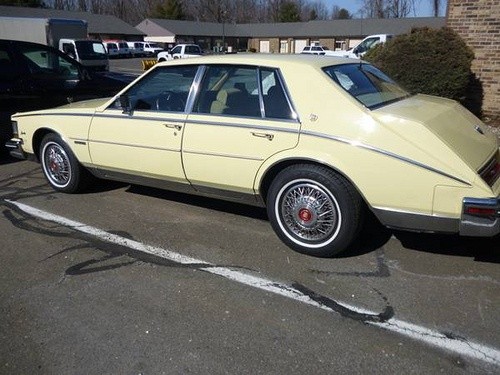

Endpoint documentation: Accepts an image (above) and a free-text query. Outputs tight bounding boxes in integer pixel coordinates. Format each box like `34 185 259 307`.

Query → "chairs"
209 86 290 120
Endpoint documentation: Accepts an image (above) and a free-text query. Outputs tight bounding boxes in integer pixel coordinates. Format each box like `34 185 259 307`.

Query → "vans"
101 39 164 57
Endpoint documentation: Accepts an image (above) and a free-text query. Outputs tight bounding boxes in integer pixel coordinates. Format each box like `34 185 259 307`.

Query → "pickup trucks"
157 43 237 63
325 34 392 59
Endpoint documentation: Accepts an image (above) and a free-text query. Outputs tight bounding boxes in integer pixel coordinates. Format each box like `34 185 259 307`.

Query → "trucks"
0 16 110 75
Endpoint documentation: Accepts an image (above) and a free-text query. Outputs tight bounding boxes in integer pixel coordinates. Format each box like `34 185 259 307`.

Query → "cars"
0 38 130 151
5 52 500 259
